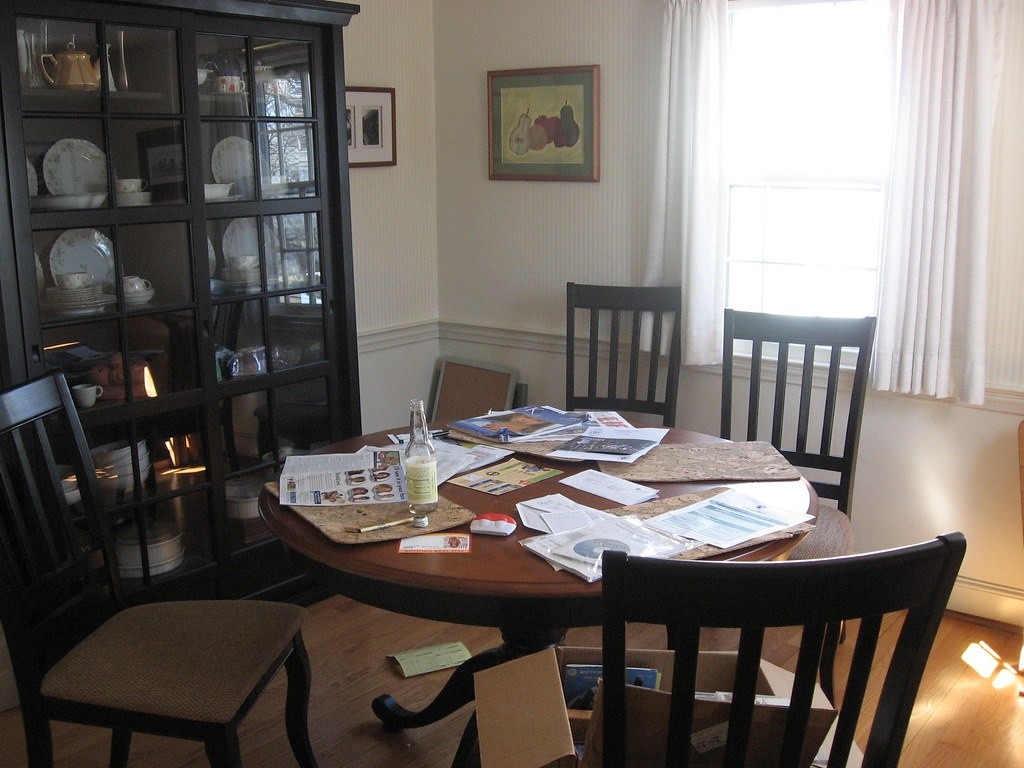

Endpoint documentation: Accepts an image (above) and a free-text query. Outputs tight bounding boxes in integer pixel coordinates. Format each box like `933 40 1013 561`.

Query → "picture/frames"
345 85 398 168
430 358 518 423
136 125 185 200
487 64 601 182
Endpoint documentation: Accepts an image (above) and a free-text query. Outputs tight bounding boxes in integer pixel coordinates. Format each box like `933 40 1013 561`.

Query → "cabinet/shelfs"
0 0 363 710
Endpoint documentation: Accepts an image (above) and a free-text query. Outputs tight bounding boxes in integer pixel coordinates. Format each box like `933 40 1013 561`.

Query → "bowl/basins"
272 346 301 368
232 345 266 374
113 524 182 567
226 495 260 520
29 192 107 210
91 438 150 493
64 486 80 506
124 288 155 304
197 70 212 84
204 184 229 200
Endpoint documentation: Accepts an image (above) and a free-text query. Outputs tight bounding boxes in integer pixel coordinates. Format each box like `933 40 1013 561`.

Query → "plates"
43 139 107 194
212 138 263 196
207 237 216 278
33 251 43 294
39 285 116 315
219 267 260 282
49 229 116 293
222 217 281 268
26 158 38 197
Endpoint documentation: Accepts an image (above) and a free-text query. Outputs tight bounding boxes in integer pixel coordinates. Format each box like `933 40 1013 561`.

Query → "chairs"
601 532 966 768
566 281 681 428
720 308 877 519
0 374 317 768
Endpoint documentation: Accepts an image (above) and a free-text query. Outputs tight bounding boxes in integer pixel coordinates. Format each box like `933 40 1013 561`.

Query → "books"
279 451 412 506
446 404 592 443
564 665 790 761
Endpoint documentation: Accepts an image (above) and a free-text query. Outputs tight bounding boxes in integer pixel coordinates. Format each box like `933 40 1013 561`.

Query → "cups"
123 275 151 295
74 384 103 407
115 178 148 193
56 270 94 290
227 255 259 271
216 75 245 94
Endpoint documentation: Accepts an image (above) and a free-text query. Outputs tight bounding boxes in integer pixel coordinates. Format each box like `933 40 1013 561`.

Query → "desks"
258 423 821 768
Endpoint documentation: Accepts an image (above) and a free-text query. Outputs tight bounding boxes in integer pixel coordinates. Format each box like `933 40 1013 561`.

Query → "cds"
573 538 630 559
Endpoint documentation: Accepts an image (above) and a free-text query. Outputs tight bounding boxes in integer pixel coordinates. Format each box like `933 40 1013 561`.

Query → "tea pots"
40 34 110 89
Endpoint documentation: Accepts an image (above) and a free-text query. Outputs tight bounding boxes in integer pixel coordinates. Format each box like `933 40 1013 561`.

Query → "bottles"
22 33 46 88
404 399 439 513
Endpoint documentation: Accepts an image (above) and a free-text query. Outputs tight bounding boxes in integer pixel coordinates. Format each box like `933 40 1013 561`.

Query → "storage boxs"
472 643 840 768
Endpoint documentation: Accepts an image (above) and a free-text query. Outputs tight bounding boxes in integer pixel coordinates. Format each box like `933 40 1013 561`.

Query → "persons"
472 413 555 433
346 466 395 501
